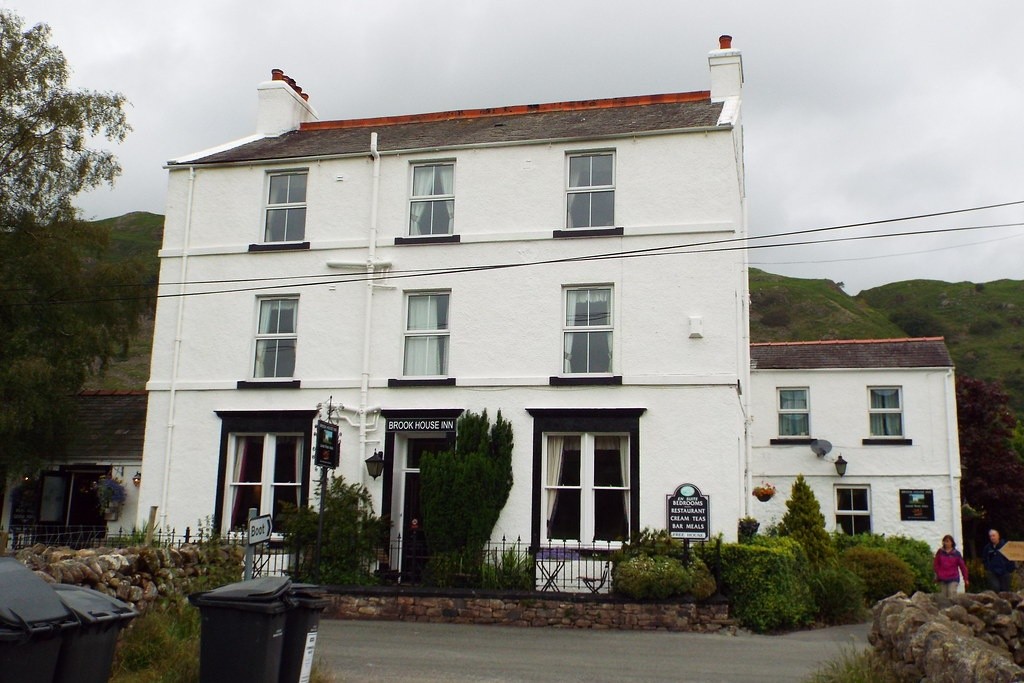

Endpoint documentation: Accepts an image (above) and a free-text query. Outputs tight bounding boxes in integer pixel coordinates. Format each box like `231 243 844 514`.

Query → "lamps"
365 447 386 480
834 455 848 477
132 471 141 486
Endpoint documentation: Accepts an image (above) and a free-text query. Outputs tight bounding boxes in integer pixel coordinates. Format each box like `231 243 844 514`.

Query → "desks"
537 560 571 592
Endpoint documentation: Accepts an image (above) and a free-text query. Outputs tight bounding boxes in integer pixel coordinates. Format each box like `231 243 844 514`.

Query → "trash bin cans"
0 559 141 683
186 576 331 683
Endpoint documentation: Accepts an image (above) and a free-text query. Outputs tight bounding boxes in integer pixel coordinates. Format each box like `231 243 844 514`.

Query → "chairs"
244 552 271 579
582 561 609 595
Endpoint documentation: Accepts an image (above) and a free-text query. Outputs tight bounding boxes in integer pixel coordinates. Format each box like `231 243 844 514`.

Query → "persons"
982 529 1015 594
933 535 969 598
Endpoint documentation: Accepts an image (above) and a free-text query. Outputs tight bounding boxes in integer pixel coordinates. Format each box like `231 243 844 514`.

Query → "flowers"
752 481 776 498
85 475 127 514
